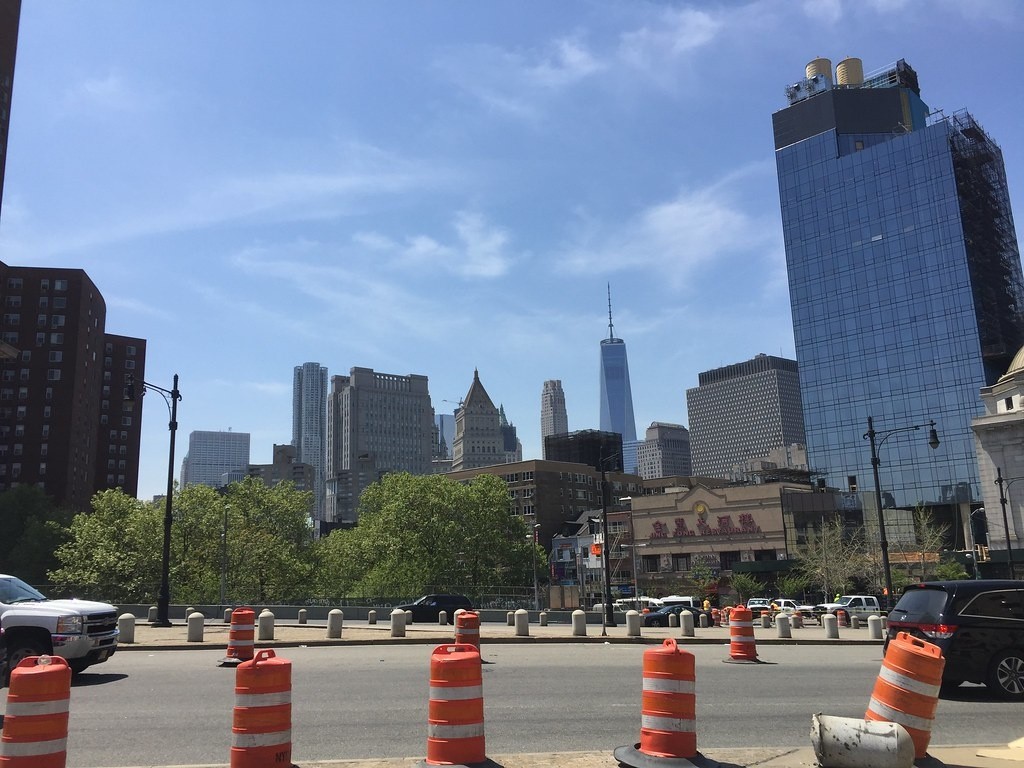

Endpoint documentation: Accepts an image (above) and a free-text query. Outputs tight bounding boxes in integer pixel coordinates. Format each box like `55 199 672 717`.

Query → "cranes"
443 397 463 407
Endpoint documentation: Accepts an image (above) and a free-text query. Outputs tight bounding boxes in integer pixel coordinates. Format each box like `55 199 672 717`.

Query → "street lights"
533 523 542 611
220 506 231 604
126 371 182 629
594 445 623 627
968 508 985 580
618 543 646 611
862 415 941 616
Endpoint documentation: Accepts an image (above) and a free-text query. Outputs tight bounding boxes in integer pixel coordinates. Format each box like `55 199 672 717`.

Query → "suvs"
747 597 771 619
883 578 1024 702
774 599 816 618
813 595 880 626
395 593 473 625
0 573 120 688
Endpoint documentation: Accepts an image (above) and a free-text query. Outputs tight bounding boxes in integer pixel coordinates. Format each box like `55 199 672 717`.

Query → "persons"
834 593 841 603
879 586 888 610
704 596 713 627
769 600 779 623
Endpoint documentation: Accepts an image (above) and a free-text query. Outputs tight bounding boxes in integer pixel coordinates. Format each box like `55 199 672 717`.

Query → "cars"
644 604 713 627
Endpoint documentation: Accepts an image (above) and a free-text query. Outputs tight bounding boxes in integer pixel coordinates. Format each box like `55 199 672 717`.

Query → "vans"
661 596 702 610
616 597 664 611
592 604 631 612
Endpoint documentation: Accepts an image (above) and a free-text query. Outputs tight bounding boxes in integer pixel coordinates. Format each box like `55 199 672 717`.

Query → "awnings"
731 559 801 573
